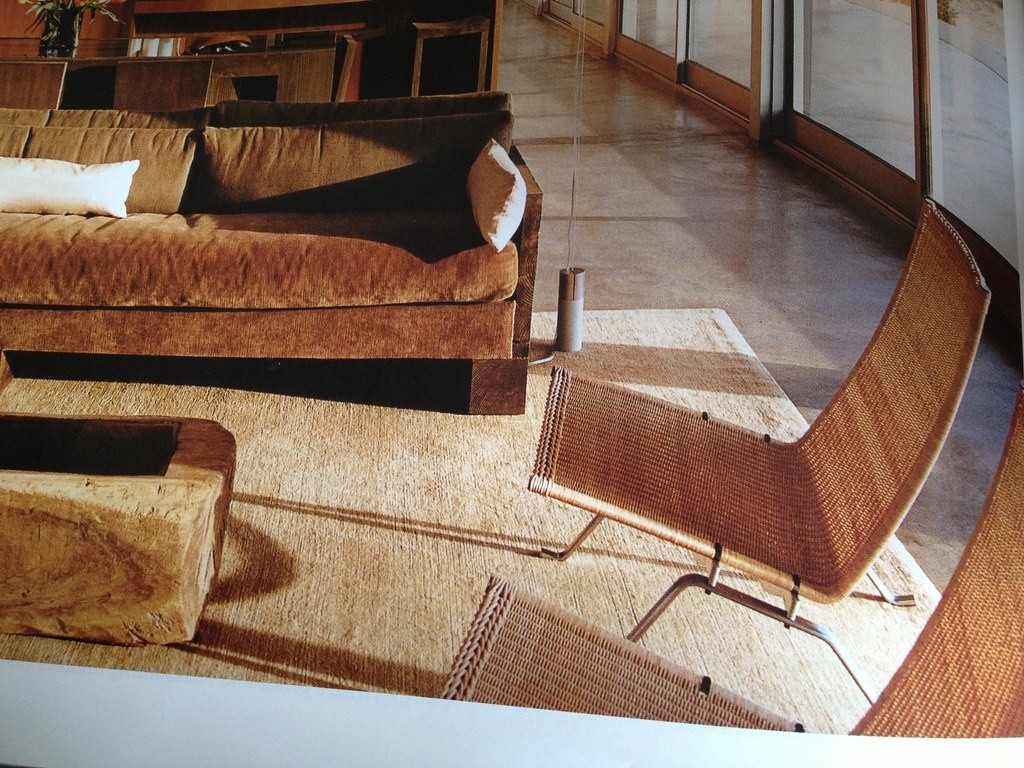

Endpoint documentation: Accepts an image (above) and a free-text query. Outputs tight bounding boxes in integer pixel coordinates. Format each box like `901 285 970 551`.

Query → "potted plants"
17 0 127 59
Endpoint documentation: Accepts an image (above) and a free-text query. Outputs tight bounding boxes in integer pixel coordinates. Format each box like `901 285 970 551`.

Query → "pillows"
0 157 141 219
466 137 528 253
0 123 199 215
199 108 519 216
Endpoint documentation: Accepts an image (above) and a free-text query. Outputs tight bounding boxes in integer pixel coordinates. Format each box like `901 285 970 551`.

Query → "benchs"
0 90 542 415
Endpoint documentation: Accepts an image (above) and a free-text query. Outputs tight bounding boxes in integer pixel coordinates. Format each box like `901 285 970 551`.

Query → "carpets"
1 304 945 737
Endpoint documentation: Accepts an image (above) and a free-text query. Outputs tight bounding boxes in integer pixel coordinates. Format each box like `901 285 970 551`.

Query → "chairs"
437 382 1023 738
524 196 994 707
